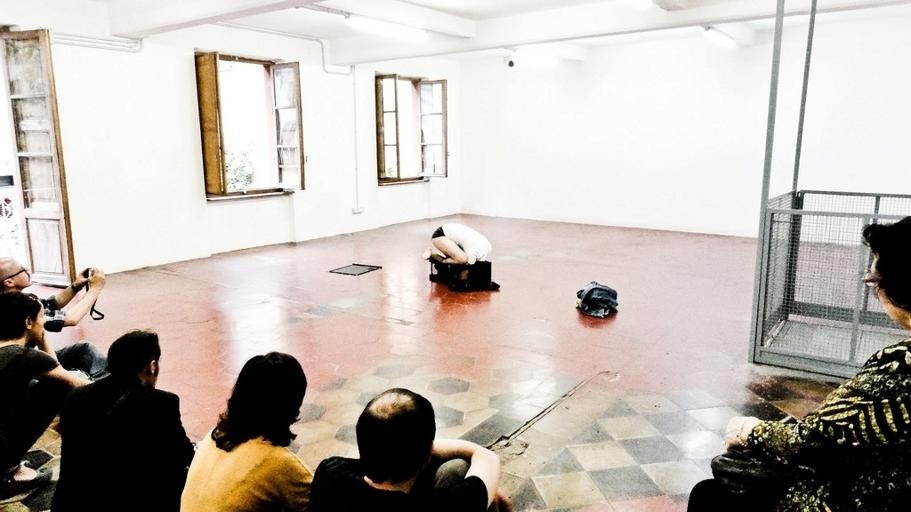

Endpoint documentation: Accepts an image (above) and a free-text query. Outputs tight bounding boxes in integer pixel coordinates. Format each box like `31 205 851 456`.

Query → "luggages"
433 257 493 291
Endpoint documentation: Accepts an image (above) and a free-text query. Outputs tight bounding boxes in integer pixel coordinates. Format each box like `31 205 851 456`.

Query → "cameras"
84 269 93 278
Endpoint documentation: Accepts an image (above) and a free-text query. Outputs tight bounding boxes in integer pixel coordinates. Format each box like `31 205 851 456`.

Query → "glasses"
860 272 885 286
2 266 27 286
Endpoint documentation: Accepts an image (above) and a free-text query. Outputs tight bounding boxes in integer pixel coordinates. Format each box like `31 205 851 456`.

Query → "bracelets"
72 282 82 292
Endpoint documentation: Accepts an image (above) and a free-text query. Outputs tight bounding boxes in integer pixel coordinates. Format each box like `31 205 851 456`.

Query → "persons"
46 327 199 512
422 221 494 293
680 214 911 512
179 349 319 512
299 385 514 512
0 254 113 386
1 291 97 495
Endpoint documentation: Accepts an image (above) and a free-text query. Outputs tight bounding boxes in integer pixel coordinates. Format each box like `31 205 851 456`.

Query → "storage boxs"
438 260 492 292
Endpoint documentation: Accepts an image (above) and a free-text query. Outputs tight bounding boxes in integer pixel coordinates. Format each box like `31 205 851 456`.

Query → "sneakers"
0 466 53 488
